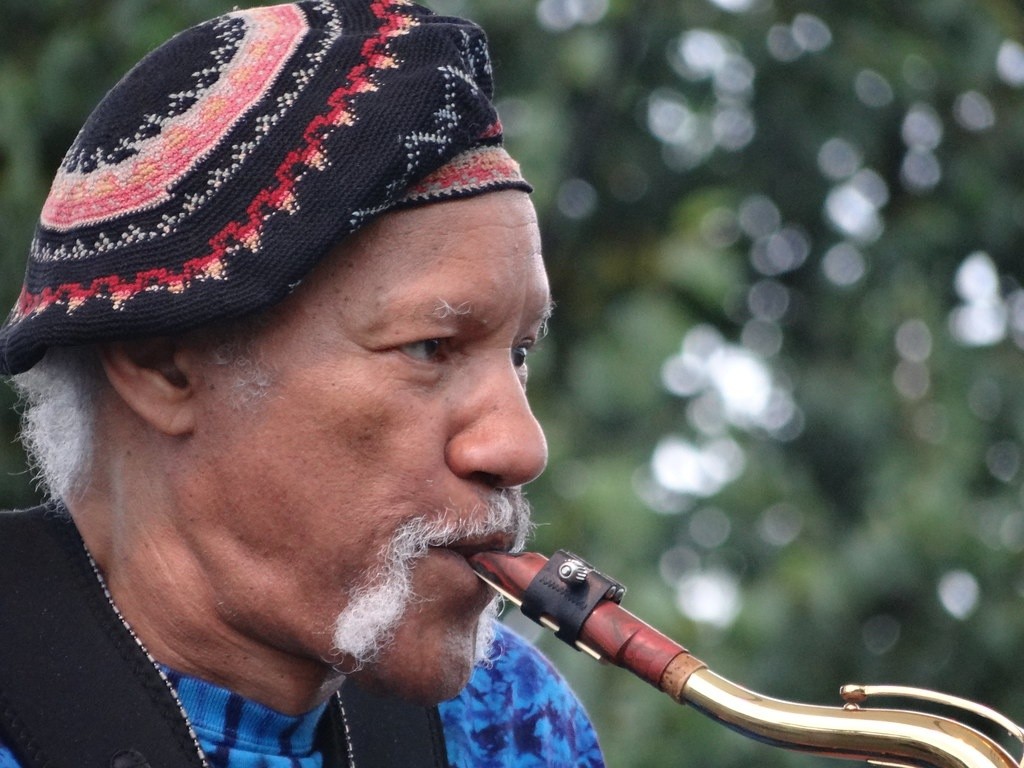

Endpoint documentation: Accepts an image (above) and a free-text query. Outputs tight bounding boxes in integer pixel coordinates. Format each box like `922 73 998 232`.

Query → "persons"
0 0 604 768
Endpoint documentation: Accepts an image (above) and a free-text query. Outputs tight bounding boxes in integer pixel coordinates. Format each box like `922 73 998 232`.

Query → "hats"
0 0 534 377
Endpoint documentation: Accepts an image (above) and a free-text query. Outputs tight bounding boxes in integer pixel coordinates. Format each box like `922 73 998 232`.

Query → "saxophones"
465 547 1023 767
